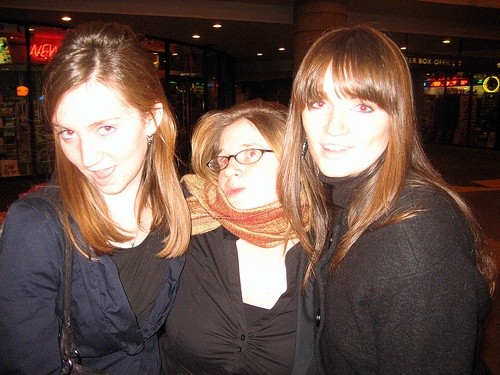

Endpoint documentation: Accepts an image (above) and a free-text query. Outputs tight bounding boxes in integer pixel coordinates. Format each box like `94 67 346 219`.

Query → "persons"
157 99 321 375
276 23 497 375
0 22 191 375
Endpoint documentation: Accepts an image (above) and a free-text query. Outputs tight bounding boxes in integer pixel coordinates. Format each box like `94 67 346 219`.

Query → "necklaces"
121 224 140 248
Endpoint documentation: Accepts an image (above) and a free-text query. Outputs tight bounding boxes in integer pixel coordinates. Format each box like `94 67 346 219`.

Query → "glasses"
206 149 274 172
